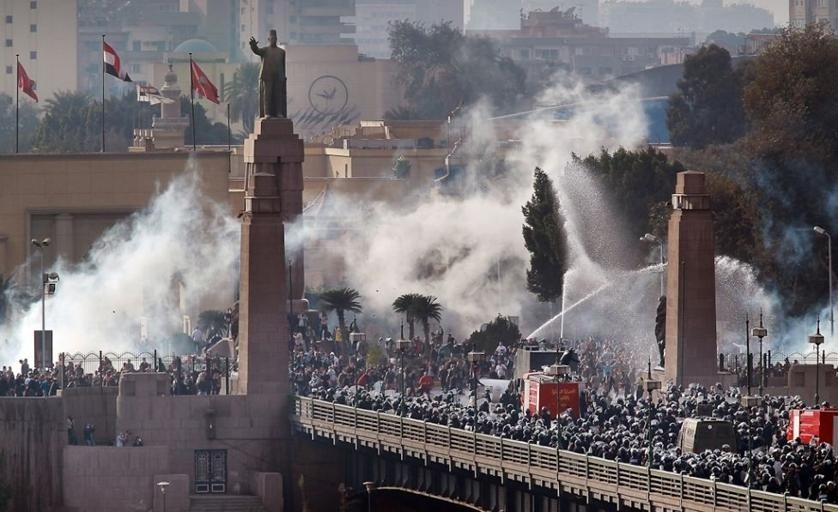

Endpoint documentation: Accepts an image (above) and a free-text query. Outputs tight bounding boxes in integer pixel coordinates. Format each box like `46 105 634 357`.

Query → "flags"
102 40 135 82
17 61 40 102
190 59 221 106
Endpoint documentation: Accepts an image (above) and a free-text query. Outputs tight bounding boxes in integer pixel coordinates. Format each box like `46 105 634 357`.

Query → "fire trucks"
515 370 583 415
784 408 837 463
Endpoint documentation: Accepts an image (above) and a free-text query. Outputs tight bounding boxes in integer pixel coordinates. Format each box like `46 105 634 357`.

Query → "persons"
65 414 77 445
115 429 144 447
0 352 225 400
249 28 290 122
83 421 97 446
190 325 204 353
291 308 838 505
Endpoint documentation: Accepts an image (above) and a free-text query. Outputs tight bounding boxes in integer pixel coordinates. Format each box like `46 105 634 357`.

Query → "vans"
673 414 737 456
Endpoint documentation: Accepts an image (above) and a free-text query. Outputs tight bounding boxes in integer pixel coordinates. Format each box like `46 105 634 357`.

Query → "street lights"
347 312 368 406
811 223 835 338
224 312 233 395
467 341 486 432
808 312 825 403
157 481 170 512
740 393 763 491
30 235 53 373
394 316 412 415
363 481 376 511
752 307 768 394
641 354 665 468
644 232 664 297
287 256 298 394
540 345 575 446
